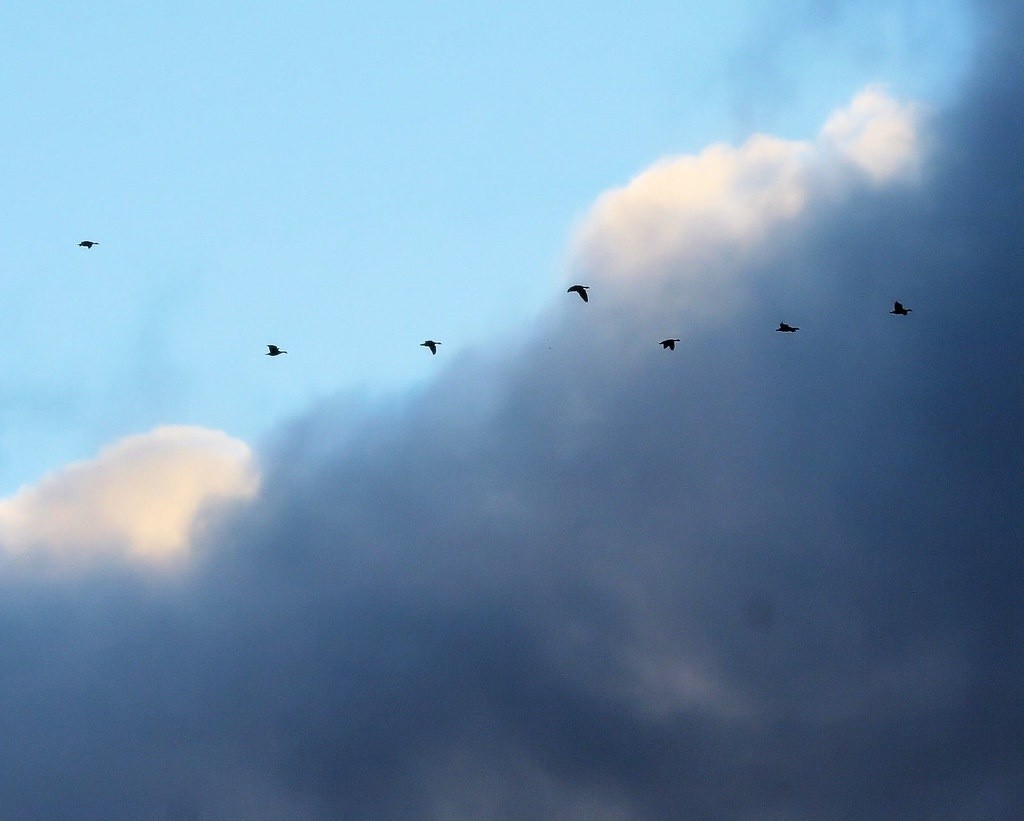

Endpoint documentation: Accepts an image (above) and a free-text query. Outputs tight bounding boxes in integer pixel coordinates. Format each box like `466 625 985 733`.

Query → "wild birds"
889 300 913 316
659 338 680 351
420 340 442 355
775 322 799 333
567 284 590 303
77 241 99 249
265 344 287 356
